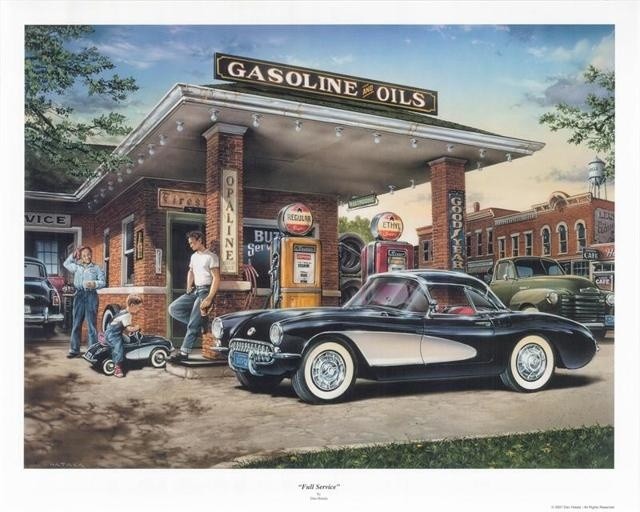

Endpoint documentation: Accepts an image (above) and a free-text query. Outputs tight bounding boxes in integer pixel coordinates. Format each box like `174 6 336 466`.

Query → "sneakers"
66 352 79 359
164 351 189 363
114 365 124 378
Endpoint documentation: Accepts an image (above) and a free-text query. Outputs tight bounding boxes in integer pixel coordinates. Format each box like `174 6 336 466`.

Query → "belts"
195 285 210 289
